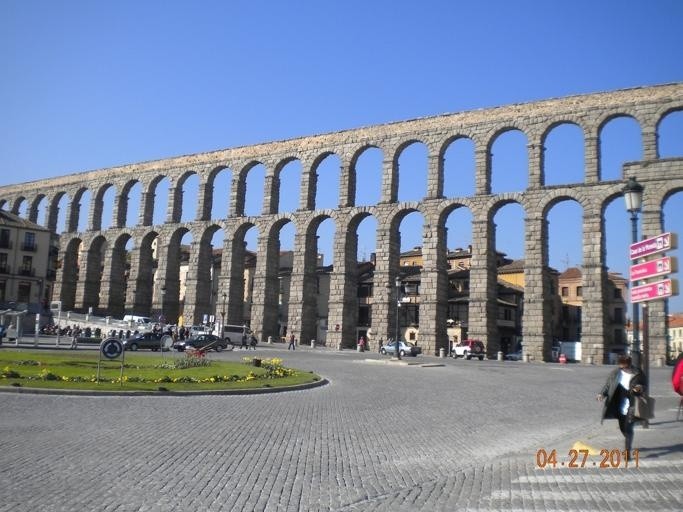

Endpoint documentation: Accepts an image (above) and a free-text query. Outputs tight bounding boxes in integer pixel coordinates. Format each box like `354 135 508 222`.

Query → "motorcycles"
39 322 82 338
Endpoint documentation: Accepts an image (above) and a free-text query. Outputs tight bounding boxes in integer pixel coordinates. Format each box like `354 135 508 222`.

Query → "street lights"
218 292 226 330
391 276 401 362
619 175 644 426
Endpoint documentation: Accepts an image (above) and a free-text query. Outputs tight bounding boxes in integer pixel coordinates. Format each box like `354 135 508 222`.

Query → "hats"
618 355 632 364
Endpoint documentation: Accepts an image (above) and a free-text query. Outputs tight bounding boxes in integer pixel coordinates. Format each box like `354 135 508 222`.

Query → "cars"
503 350 522 361
122 332 168 352
174 334 227 353
380 341 420 357
122 315 212 338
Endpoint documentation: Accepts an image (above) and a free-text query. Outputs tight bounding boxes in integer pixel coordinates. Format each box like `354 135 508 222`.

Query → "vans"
211 323 258 346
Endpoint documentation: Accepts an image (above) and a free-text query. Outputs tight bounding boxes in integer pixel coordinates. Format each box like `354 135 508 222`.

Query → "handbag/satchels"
634 394 655 418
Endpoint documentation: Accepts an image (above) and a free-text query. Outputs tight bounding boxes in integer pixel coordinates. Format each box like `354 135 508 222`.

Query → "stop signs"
334 324 339 330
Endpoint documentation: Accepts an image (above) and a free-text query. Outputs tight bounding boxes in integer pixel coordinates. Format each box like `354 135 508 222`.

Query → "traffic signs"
629 280 671 304
629 233 671 261
629 257 670 281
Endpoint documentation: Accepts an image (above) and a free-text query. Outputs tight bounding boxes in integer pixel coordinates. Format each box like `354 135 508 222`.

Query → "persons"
671 352 683 398
595 352 650 463
357 335 401 356
287 334 297 349
0 317 258 354
